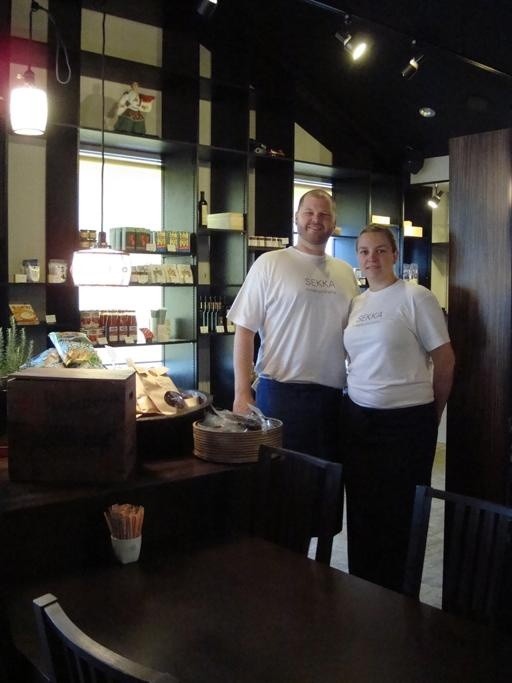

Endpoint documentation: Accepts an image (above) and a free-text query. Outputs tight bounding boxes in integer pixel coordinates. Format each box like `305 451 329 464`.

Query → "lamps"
10 0 71 136
73 0 133 287
335 13 437 80
428 183 443 208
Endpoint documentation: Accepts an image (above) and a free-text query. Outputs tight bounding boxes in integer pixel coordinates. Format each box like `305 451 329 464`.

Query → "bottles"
197 295 236 334
100 309 137 344
197 191 208 229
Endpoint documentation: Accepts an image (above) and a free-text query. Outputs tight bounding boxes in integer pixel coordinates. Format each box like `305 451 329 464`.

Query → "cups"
110 534 142 563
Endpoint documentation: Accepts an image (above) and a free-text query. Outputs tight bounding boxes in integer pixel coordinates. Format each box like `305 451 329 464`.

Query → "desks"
0 439 256 588
4 523 512 683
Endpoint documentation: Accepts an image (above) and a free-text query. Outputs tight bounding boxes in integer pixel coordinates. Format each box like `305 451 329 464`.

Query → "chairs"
256 443 345 566
32 592 178 683
401 483 512 630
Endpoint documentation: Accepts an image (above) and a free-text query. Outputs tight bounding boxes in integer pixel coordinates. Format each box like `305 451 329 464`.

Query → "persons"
105 77 157 134
222 184 363 564
339 219 458 606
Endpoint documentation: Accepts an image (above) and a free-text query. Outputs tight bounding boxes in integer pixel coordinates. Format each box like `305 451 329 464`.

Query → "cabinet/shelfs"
78 8 434 390
0 0 433 412
